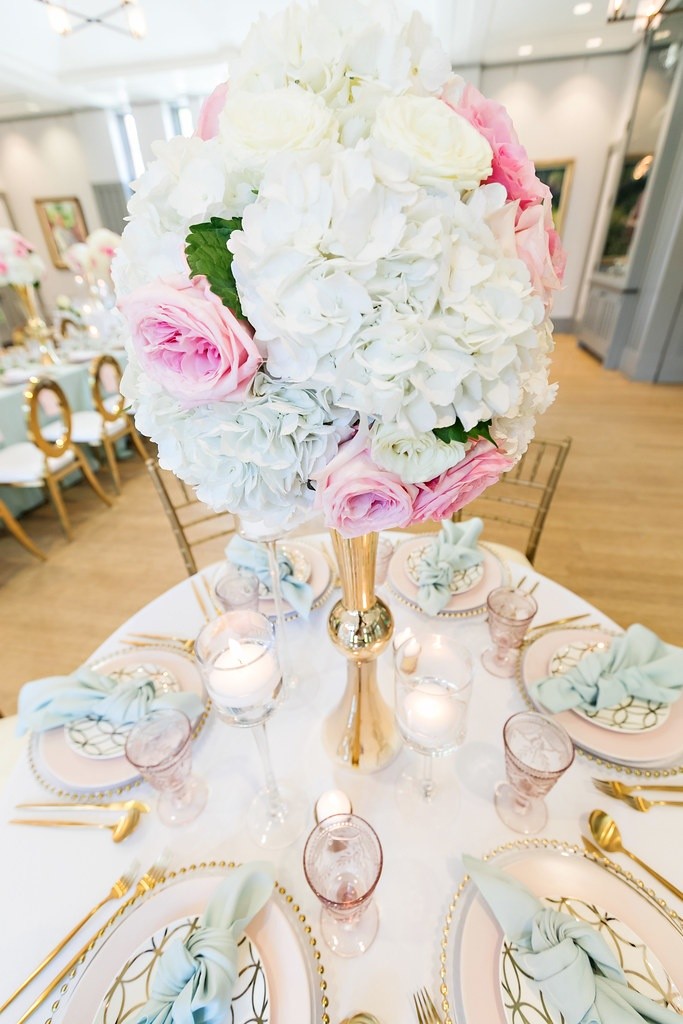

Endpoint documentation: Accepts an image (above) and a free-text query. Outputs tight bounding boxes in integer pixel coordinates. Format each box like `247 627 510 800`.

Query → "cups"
215 571 260 611
373 538 392 589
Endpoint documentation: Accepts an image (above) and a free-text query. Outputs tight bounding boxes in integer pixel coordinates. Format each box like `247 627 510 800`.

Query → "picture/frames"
35 197 89 269
533 158 574 242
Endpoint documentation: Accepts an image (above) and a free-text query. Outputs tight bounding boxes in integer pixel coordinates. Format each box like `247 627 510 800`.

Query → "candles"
214 628 457 828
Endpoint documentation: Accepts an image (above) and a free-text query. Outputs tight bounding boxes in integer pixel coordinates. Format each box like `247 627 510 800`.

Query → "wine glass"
125 708 208 826
481 586 537 678
393 632 474 830
192 612 310 849
303 817 385 959
493 711 575 835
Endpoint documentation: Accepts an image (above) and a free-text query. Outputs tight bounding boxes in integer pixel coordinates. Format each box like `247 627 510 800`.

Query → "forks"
590 776 683 813
485 576 539 622
1 855 175 1024
413 988 442 1024
123 632 196 648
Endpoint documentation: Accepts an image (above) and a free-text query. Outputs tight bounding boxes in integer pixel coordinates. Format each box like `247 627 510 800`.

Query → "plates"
63 662 183 759
405 544 484 594
548 640 671 734
66 352 99 362
216 540 336 628
45 861 330 1024
384 534 509 619
440 839 683 1024
0 373 33 385
28 643 213 802
515 627 683 769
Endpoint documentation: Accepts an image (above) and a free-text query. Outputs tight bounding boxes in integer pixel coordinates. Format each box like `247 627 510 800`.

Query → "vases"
14 285 49 346
320 529 403 773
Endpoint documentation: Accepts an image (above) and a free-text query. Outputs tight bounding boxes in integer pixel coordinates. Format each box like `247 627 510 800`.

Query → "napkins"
224 533 314 621
414 517 483 616
126 858 279 1024
461 848 683 1024
534 624 681 716
14 670 207 741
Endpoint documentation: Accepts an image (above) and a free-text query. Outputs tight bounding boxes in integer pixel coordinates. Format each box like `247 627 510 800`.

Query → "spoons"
589 809 683 901
9 808 140 844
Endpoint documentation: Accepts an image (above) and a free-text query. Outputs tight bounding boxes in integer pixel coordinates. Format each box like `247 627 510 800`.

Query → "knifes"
15 801 150 813
581 836 683 930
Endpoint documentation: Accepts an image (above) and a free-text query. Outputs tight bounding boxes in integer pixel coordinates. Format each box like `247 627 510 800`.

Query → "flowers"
110 2 558 538
0 229 44 287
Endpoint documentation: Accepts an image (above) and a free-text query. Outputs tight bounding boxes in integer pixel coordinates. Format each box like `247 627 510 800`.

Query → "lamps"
606 0 683 42
36 0 146 42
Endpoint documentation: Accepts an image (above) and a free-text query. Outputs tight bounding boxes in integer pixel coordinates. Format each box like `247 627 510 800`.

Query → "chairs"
0 376 114 542
452 435 576 565
0 354 150 561
146 454 259 576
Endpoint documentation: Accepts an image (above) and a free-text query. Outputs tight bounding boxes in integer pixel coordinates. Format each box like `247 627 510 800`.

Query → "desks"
0 529 683 1024
0 347 133 524
